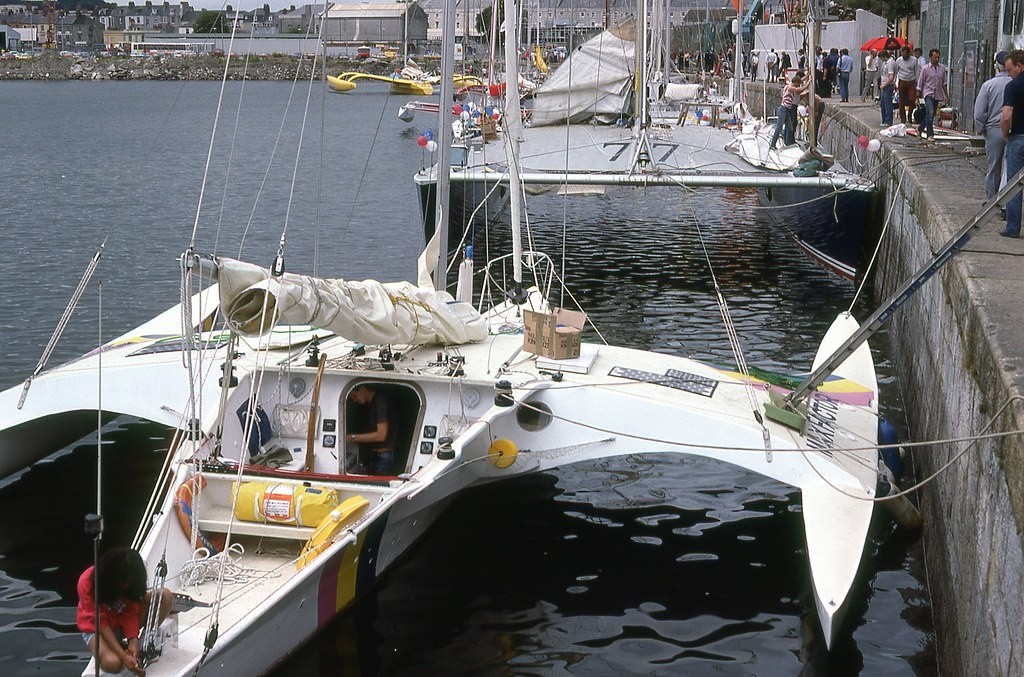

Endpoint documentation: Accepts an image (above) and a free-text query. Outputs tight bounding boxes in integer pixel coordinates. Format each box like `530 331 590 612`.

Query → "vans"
384 52 397 58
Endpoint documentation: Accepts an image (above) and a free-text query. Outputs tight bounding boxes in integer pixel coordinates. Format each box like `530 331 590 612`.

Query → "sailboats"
0 1 881 677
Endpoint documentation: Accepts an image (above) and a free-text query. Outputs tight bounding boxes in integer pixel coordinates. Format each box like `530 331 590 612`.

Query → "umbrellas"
860 36 914 51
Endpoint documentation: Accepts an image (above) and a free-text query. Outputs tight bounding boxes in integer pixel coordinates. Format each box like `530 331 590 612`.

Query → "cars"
376 53 385 59
209 49 349 60
2 51 111 59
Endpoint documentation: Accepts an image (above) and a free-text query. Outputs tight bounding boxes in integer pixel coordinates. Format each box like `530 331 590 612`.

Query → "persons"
541 48 566 63
346 383 398 476
770 67 825 150
972 48 1024 238
670 47 745 80
750 51 759 82
861 47 927 127
76 547 172 677
765 45 853 102
914 48 949 142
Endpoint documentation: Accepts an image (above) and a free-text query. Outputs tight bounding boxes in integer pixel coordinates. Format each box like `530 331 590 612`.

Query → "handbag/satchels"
838 57 842 76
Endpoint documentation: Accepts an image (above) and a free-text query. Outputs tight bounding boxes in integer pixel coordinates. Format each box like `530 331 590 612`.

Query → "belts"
370 448 394 453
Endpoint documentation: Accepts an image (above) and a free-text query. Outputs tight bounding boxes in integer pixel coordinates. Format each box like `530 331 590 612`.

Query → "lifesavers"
295 494 372 573
174 473 227 558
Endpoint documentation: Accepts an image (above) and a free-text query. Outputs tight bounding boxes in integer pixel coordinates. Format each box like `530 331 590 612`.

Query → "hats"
997 51 1008 65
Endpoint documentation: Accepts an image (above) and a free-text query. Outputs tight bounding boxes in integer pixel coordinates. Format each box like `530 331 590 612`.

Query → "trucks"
357 47 371 59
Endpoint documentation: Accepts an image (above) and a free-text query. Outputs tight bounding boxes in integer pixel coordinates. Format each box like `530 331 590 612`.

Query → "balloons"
418 129 437 152
454 102 500 124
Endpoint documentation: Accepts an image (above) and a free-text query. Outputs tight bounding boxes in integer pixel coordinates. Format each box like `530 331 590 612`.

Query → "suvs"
555 46 568 56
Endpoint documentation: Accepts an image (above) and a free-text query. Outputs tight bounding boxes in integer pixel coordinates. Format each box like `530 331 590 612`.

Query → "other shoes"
999 229 1019 238
125 643 159 662
861 96 866 102
916 128 922 140
770 145 777 150
880 123 892 126
1000 209 1006 221
927 136 936 142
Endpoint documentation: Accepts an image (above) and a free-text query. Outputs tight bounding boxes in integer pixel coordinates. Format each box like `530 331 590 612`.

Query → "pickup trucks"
423 53 442 60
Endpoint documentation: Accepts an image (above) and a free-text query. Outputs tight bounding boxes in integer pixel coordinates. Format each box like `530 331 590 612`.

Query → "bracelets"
351 434 356 442
128 637 139 644
